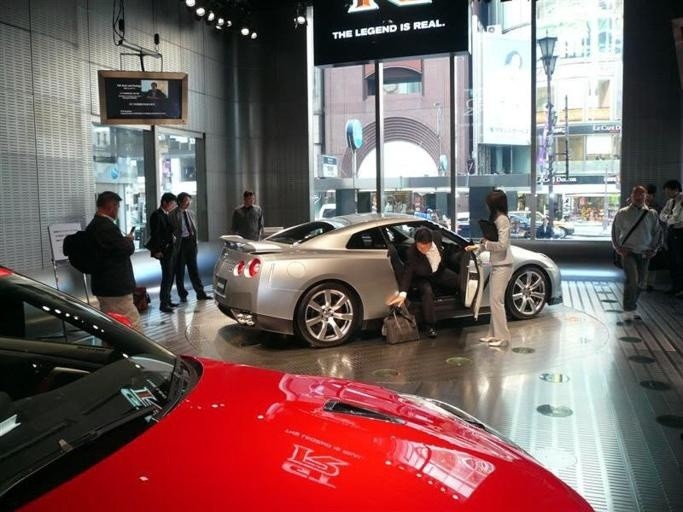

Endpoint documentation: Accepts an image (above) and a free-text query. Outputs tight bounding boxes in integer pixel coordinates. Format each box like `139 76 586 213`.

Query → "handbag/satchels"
611 247 622 268
382 307 420 344
143 235 169 257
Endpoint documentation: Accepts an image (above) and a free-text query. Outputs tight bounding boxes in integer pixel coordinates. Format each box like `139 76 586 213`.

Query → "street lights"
535 26 565 226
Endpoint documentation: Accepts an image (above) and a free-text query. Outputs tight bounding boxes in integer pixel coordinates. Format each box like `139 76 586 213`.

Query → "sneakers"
181 297 186 302
203 294 213 299
623 310 634 321
633 311 640 318
170 303 179 307
160 304 174 311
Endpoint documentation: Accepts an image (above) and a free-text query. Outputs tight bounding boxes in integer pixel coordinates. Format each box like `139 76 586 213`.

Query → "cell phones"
130 227 135 235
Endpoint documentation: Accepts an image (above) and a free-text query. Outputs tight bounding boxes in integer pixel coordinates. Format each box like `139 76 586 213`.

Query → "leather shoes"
480 336 494 342
424 327 440 338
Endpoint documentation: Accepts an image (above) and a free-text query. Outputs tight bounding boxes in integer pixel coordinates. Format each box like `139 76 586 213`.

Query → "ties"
183 210 192 234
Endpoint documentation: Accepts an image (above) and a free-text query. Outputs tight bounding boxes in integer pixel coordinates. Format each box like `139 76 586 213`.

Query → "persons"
83 190 142 346
464 189 514 347
231 191 264 236
610 178 683 322
510 198 550 239
145 82 167 99
147 193 181 313
169 192 214 302
387 229 458 338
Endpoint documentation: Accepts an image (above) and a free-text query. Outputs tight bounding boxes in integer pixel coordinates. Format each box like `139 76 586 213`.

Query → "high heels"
488 339 512 346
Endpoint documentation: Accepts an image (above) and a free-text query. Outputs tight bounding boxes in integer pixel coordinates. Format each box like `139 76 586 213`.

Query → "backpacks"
63 231 100 274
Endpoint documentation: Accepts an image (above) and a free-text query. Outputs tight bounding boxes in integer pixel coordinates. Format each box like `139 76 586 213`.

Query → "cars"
447 212 565 239
214 210 562 346
510 210 574 238
0 265 599 512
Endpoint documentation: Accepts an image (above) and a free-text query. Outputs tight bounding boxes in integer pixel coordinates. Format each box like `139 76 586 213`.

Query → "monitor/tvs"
98 70 188 125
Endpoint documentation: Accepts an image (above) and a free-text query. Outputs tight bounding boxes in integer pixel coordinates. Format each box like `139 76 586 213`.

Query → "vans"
319 203 377 220
